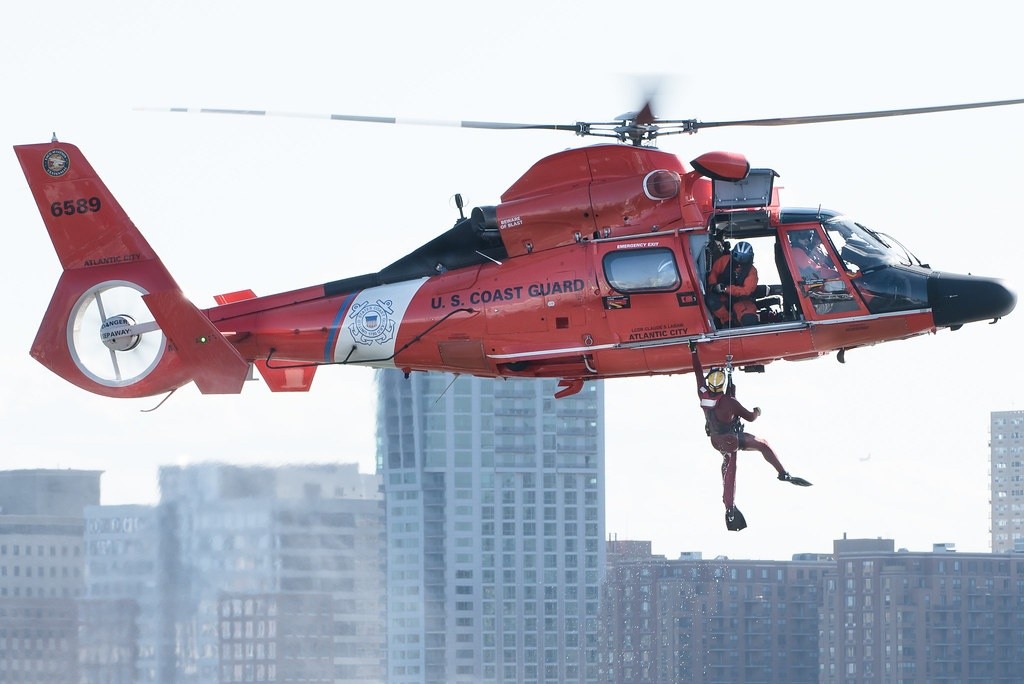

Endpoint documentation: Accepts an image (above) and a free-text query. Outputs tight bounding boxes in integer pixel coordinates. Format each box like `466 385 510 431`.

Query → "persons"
788 228 892 313
688 339 812 531
704 242 760 328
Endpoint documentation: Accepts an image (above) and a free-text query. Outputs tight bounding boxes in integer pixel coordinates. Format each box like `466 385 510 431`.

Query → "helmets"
732 242 754 265
789 229 810 242
708 371 725 393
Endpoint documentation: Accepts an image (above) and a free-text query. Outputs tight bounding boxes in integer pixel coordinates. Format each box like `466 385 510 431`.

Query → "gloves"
713 284 727 295
689 338 698 352
754 407 761 416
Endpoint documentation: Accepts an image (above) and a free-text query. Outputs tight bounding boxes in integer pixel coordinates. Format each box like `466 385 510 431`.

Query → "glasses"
798 234 810 240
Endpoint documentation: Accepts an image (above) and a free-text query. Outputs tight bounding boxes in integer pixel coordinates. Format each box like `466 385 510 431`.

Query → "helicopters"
11 97 1023 402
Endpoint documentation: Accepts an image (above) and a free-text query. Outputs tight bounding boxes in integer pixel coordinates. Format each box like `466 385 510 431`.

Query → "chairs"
707 233 768 314
772 232 802 308
